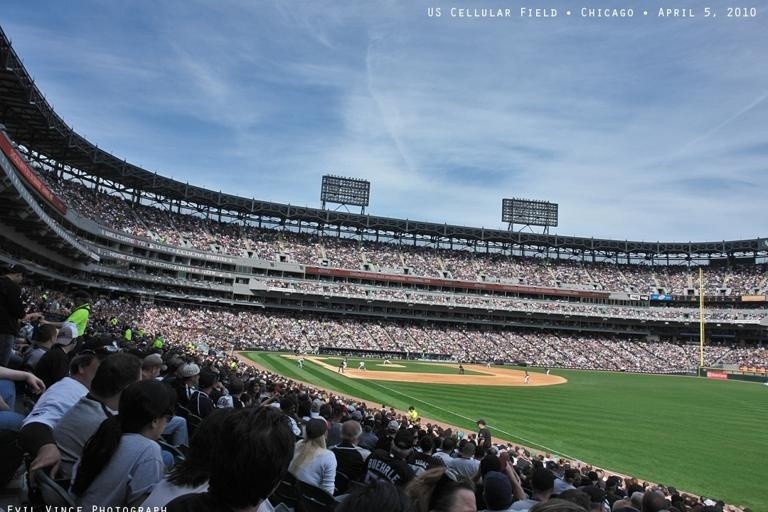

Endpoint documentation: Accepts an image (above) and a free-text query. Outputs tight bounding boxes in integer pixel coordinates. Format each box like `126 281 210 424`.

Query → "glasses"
90 345 124 354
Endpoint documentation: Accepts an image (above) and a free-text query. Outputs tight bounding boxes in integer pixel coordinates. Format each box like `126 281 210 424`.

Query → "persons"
0 135 766 374
523 370 529 383
0 375 420 511
420 423 751 512
476 419 491 449
544 368 549 375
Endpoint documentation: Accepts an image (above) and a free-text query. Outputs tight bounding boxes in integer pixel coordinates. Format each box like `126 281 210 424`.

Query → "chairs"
23 452 78 511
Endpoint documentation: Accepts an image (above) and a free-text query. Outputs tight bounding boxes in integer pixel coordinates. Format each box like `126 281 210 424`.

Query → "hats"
393 429 414 450
55 326 79 346
182 363 201 377
306 415 332 434
